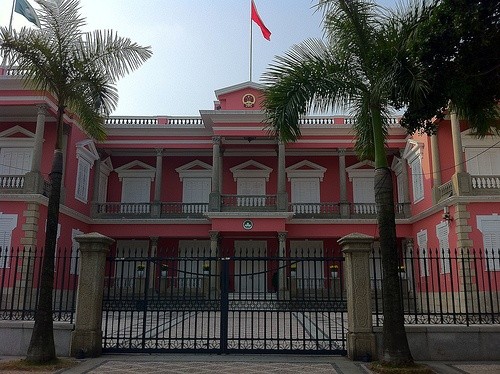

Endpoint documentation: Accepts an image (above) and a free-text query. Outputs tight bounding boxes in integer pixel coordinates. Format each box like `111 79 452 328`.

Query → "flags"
251 2 272 42
